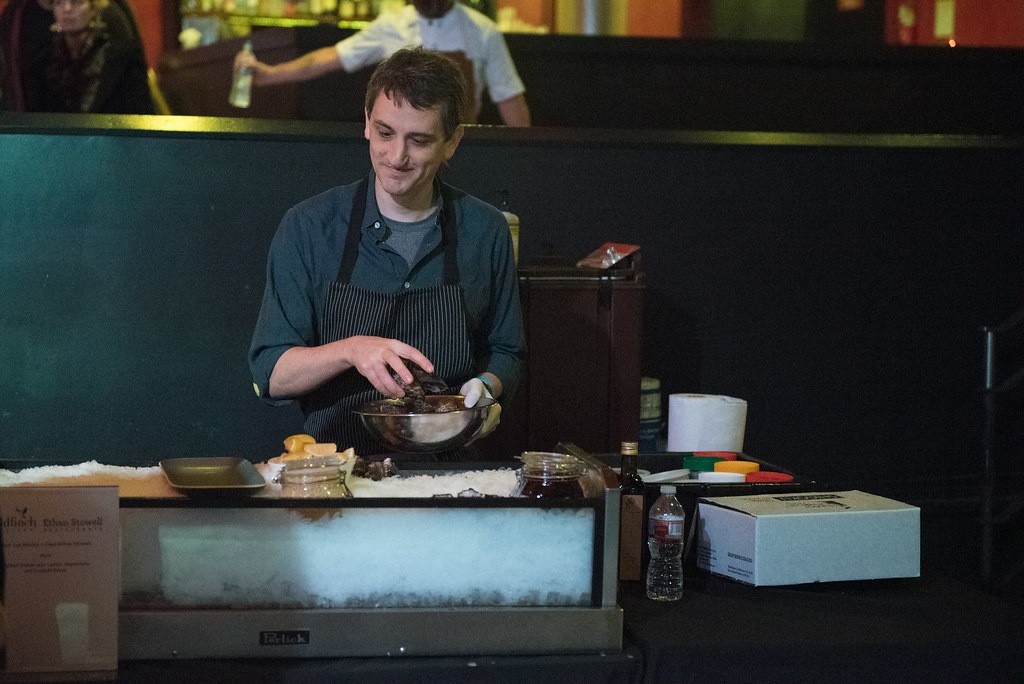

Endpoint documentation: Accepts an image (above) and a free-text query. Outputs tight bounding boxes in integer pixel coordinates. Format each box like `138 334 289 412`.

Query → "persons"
232 0 531 127
248 44 527 462
18 0 152 115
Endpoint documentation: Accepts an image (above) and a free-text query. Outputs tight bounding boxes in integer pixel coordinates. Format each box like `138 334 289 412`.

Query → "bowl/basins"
350 395 498 455
268 454 358 480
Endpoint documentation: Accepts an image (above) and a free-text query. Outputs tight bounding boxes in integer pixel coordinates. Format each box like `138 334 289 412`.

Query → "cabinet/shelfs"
481 261 653 461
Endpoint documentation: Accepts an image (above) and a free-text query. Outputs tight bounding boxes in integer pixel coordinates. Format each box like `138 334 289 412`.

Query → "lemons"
280 435 347 465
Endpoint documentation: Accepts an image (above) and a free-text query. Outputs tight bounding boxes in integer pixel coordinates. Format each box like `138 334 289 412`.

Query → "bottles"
618 442 648 582
646 485 685 602
228 39 256 108
283 456 354 518
509 451 588 500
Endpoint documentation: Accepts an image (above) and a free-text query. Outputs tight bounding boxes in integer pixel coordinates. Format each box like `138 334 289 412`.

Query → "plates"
158 457 266 492
581 241 641 269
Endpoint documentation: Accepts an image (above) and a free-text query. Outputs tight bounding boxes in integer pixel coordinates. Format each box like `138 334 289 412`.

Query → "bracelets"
481 381 494 397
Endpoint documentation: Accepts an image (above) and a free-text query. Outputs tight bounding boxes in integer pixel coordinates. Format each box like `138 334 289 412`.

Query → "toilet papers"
666 391 750 453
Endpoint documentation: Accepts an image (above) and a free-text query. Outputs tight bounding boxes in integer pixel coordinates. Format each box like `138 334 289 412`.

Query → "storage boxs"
693 491 921 586
0 485 120 671
